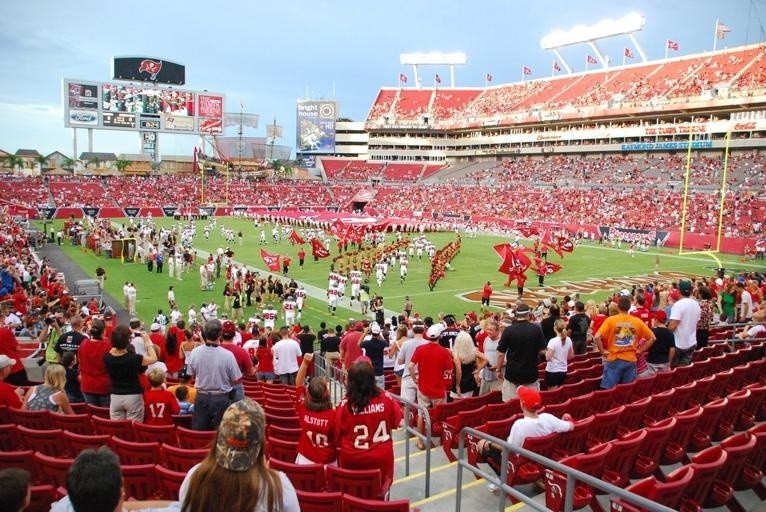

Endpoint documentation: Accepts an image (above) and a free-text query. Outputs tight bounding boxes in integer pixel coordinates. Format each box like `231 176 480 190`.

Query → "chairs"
333 325 766 512
0 376 409 512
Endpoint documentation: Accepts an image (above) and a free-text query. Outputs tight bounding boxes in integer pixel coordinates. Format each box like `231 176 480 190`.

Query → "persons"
327 43 765 189
103 85 193 129
1 174 765 512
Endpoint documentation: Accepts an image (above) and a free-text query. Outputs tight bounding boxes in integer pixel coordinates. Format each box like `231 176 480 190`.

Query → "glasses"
206 343 218 347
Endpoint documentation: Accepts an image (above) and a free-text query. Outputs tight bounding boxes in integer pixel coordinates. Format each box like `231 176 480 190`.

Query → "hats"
355 322 380 335
0 355 16 368
518 386 543 412
427 325 440 338
151 322 178 336
223 321 235 334
679 281 691 291
651 309 667 322
215 398 265 472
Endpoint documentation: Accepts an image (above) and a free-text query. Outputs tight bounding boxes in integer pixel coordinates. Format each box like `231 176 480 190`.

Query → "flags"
715 20 731 39
